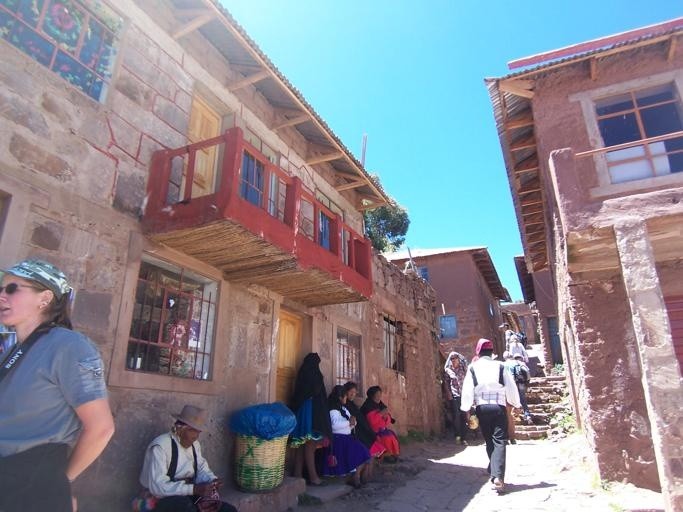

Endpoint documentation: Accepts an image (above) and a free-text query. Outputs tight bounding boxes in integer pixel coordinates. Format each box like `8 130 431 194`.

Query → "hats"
499 323 510 329
171 404 209 432
0 259 71 302
476 339 493 355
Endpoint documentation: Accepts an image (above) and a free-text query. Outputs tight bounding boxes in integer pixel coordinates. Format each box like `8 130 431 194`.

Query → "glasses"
0 282 45 295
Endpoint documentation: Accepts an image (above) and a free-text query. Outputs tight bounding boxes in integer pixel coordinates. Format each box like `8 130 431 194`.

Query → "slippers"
494 480 505 493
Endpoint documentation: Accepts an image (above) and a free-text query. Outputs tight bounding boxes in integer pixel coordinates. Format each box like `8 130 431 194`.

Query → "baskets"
234 433 289 491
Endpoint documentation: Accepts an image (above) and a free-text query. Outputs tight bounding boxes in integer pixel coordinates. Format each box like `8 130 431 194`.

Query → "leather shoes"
302 472 310 480
311 480 329 487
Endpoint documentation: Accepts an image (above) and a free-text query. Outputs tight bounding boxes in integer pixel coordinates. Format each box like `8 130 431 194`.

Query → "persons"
508 333 531 365
361 386 401 463
321 385 372 488
460 337 522 491
344 381 377 447
499 322 527 357
137 404 238 512
503 351 534 426
288 353 329 487
443 350 467 448
0 258 116 511
